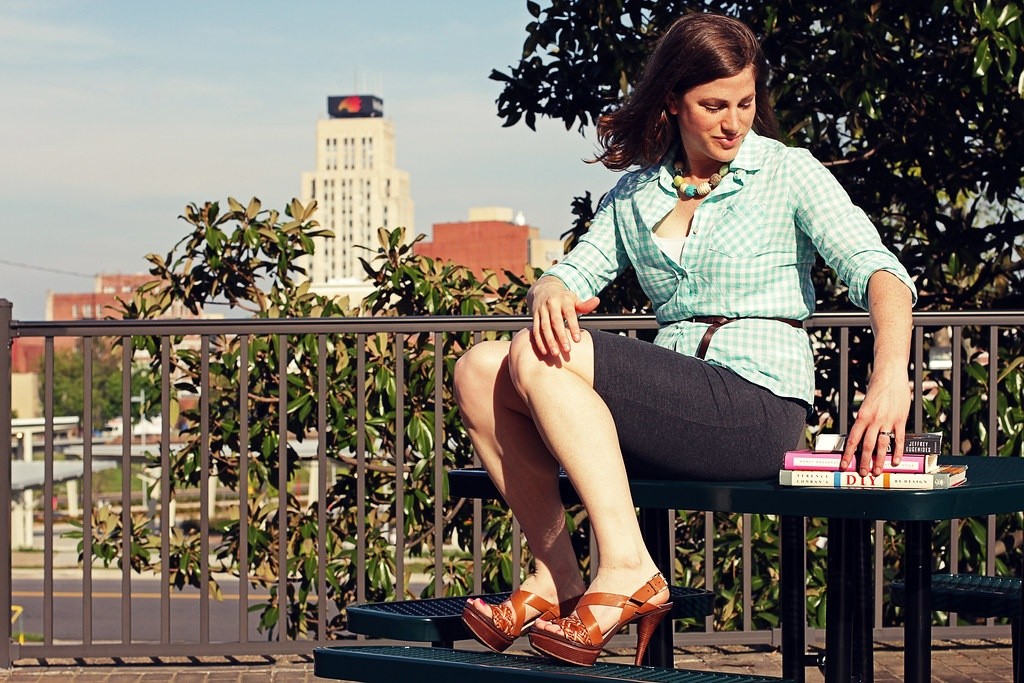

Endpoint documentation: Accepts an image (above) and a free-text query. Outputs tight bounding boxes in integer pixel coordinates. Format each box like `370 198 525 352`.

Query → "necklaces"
672 161 728 198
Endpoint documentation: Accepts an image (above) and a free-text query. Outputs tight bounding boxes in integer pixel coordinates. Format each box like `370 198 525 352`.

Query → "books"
779 432 968 490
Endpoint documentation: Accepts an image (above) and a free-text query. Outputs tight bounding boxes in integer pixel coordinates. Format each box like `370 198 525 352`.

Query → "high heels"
461 584 590 653
528 572 674 667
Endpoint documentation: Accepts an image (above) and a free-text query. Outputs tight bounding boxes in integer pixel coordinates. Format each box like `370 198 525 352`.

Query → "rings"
880 432 892 436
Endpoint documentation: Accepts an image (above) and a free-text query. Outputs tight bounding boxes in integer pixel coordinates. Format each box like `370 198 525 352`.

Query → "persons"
454 13 917 669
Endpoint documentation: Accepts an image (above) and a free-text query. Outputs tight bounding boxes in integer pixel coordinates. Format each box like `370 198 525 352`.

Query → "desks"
449 454 1023 683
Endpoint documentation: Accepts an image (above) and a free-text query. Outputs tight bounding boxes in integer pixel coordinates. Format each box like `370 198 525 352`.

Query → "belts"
686 315 803 360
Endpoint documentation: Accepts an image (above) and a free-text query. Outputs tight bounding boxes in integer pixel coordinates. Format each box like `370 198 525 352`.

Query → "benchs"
346 586 717 666
313 645 803 683
889 569 1023 683
